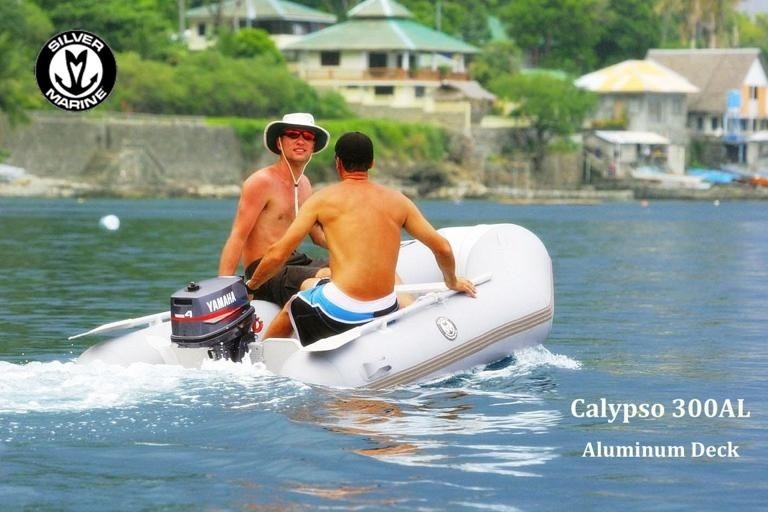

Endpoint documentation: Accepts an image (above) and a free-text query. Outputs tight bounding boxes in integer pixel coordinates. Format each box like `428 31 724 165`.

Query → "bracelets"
244 278 258 294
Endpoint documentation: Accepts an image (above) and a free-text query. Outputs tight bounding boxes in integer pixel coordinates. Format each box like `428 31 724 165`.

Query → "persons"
218 112 416 309
245 130 476 339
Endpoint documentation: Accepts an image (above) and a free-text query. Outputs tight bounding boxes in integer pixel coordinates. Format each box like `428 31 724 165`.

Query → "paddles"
68 311 171 340
299 277 492 352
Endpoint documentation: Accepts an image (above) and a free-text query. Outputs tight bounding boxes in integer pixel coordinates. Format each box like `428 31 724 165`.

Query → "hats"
263 112 331 157
334 131 373 163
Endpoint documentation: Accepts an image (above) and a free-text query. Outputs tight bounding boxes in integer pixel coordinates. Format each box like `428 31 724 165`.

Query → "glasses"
280 128 317 140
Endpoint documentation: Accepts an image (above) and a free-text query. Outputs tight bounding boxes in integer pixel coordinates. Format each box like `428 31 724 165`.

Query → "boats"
65 218 559 398
629 162 768 190
